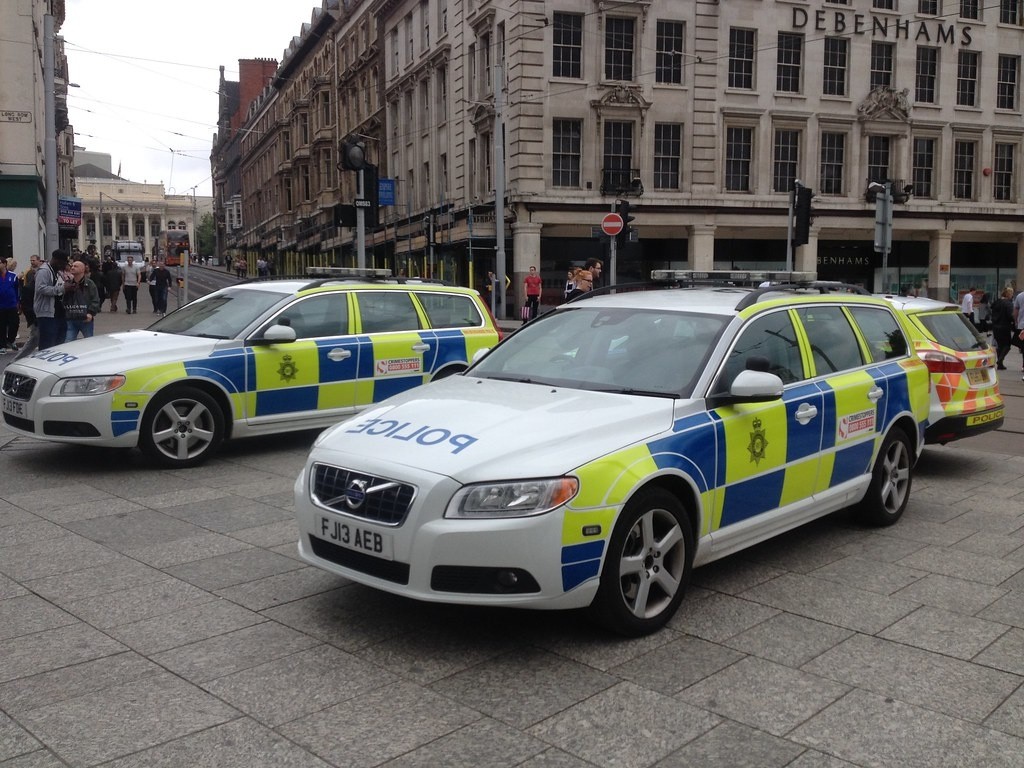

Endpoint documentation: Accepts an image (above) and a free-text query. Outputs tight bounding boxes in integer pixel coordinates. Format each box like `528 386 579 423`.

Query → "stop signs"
601 214 623 235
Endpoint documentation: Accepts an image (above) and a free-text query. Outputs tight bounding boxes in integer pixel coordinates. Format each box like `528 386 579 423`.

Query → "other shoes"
126 309 136 314
15 334 20 338
996 360 1007 370
110 305 117 313
157 312 166 317
987 333 992 337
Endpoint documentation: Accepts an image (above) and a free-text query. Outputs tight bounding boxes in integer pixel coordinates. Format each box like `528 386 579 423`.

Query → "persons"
61 260 100 343
234 254 248 280
483 269 492 309
224 251 233 272
564 258 603 303
521 266 542 326
961 286 976 328
978 294 992 337
146 260 172 318
33 248 76 351
0 254 46 355
858 85 912 117
256 256 268 281
122 256 141 315
1013 291 1024 381
991 287 1015 370
397 268 406 277
69 245 122 313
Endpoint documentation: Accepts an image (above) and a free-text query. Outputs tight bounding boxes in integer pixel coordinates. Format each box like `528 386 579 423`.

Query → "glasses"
596 267 601 270
582 278 593 283
30 260 35 262
530 269 535 270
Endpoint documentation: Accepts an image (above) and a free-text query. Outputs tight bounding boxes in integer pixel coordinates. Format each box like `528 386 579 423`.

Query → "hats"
52 248 68 261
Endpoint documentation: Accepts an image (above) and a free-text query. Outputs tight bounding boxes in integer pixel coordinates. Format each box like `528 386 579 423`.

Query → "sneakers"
0 348 7 355
8 342 18 351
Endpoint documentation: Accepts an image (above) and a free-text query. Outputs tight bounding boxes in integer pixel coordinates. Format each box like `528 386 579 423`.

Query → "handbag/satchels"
62 284 88 320
521 306 532 321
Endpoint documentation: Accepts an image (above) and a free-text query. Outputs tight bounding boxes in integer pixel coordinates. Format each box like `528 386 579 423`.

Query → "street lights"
46 82 81 262
191 185 198 251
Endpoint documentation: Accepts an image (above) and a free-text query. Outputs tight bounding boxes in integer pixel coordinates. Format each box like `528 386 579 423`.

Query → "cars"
294 271 931 636
759 279 1005 443
0 267 503 470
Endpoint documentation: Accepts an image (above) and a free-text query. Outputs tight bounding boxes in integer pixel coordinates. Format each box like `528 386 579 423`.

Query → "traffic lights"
344 145 367 170
615 199 635 237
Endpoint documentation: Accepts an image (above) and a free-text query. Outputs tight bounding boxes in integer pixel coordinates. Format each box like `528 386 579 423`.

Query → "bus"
159 230 191 266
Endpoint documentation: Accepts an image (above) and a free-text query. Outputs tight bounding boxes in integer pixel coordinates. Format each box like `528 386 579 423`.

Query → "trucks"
111 240 147 280
